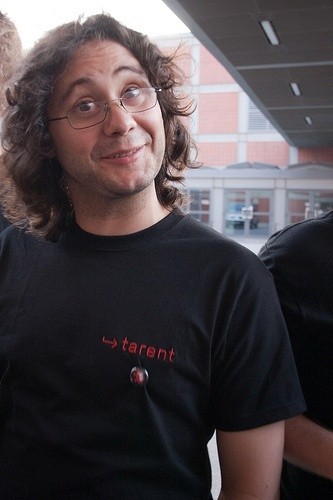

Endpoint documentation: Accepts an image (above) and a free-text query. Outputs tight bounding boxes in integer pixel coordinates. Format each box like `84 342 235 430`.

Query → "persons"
258 207 333 500
1 12 302 500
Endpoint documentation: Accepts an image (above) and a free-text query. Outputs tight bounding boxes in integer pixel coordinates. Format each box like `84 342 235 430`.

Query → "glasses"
46 83 162 130
